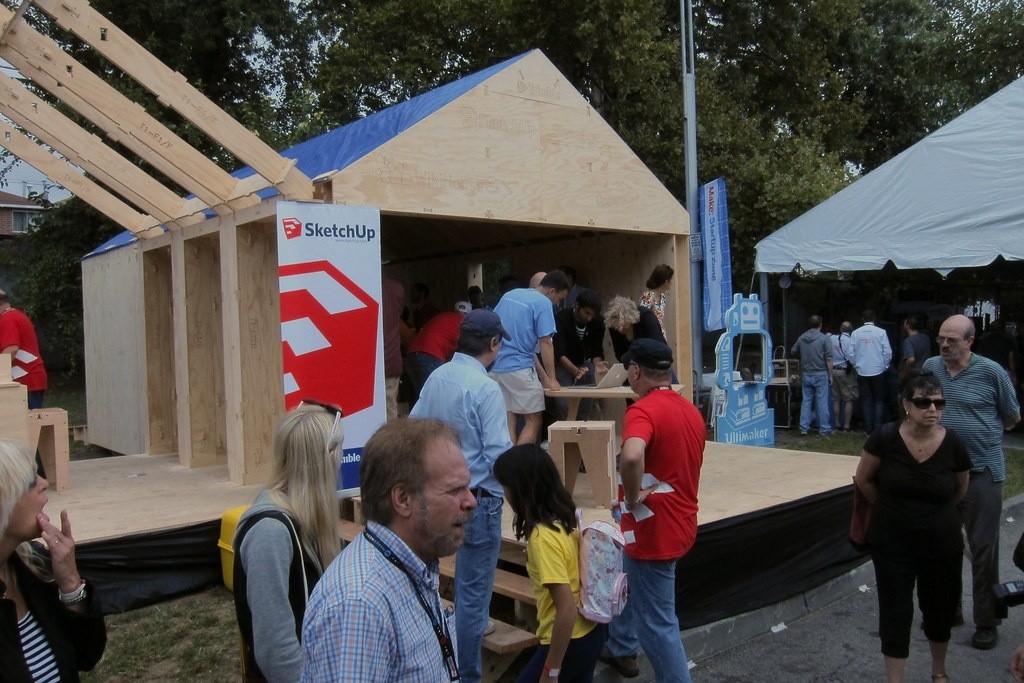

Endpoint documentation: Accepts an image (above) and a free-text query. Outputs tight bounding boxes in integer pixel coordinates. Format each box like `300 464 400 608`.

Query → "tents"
751 75 1024 375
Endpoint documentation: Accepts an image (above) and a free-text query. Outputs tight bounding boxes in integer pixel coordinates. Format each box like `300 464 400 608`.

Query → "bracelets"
58 583 85 599
543 665 561 677
623 496 640 505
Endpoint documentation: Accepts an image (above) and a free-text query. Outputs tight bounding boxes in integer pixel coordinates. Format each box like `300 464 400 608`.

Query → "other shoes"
931 673 949 683
483 620 496 637
921 614 964 629
864 431 871 438
800 431 809 435
972 623 996 649
834 426 855 433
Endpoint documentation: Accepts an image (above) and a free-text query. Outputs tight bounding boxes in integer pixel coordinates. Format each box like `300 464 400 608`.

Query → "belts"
833 367 847 370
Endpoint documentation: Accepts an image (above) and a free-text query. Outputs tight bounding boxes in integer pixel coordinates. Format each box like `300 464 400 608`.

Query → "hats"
621 339 674 370
463 308 512 342
454 301 471 314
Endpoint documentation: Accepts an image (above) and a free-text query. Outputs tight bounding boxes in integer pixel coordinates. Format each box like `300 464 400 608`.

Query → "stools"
27 406 72 493
548 420 618 509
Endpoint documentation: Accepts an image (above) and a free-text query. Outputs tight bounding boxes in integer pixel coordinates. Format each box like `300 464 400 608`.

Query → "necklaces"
916 441 923 452
0 306 11 315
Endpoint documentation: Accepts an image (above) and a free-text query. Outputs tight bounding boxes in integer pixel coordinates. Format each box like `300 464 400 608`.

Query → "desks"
0 381 28 445
544 385 686 436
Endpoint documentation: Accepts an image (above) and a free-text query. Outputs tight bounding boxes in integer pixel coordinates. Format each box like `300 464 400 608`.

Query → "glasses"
297 399 343 437
936 335 968 343
910 396 946 410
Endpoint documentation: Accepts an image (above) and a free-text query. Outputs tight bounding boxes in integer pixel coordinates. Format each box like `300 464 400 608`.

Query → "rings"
55 537 59 543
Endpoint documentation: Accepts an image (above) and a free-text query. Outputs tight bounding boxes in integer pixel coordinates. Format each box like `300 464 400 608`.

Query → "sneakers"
598 644 640 678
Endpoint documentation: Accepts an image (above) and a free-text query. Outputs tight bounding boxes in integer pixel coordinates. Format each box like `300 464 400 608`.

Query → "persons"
0 438 108 683
0 289 47 481
920 313 1020 649
600 338 706 683
380 265 679 444
792 311 1016 435
231 399 344 682
492 443 606 682
407 308 514 683
300 416 477 683
855 367 972 682
1010 532 1024 683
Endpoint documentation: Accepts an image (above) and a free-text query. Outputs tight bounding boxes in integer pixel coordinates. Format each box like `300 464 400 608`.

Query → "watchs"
59 590 86 605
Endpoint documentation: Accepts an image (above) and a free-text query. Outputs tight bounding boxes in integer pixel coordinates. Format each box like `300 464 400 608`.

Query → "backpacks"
574 507 628 624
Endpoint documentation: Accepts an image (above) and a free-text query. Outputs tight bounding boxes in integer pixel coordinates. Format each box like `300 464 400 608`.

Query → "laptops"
568 363 629 389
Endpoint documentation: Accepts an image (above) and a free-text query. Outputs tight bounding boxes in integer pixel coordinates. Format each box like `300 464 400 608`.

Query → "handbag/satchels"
847 423 898 553
